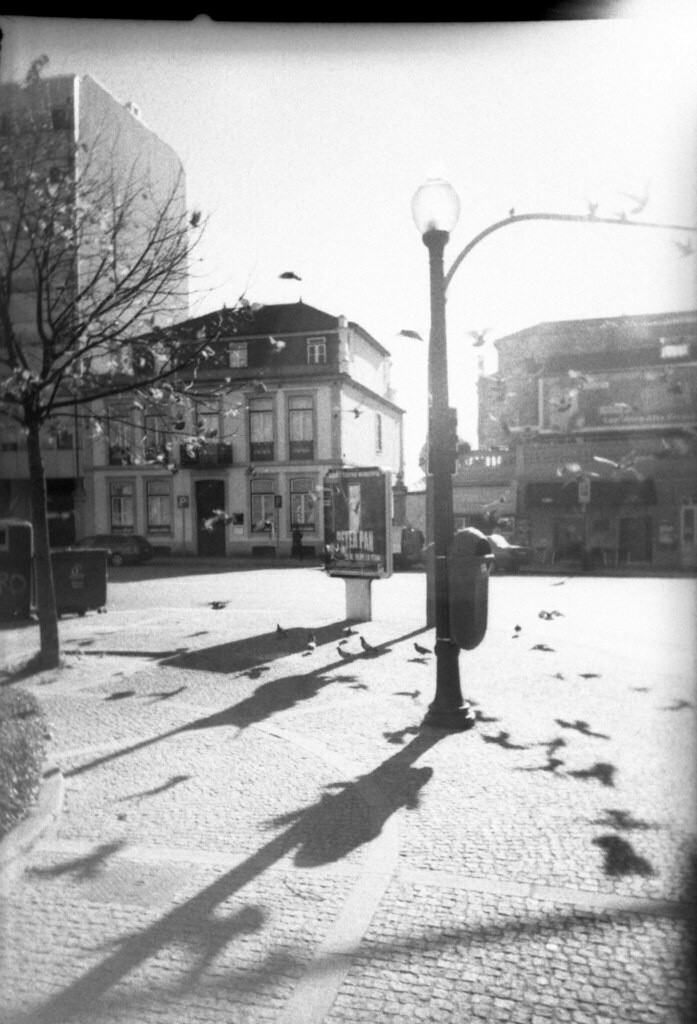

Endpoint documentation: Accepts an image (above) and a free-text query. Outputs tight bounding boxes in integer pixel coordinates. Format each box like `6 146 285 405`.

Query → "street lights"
411 179 476 729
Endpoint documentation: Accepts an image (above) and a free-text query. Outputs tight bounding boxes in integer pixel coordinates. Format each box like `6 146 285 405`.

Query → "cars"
484 535 530 573
74 534 156 566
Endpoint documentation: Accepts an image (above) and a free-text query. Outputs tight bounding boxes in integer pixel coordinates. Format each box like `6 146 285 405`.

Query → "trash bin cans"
50 548 108 621
